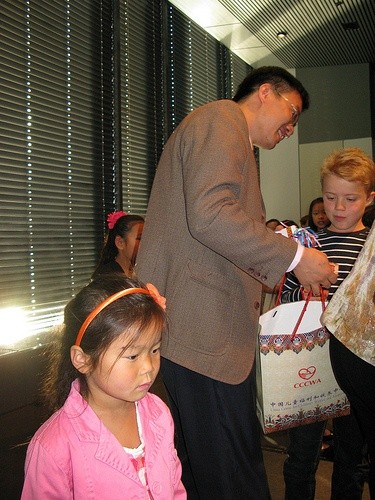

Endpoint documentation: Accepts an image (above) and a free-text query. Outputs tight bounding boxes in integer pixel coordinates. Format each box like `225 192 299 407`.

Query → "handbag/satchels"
255 273 351 434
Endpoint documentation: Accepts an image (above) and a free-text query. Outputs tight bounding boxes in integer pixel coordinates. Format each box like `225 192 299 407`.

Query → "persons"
132 66 338 500
267 197 331 231
283 148 375 500
21 274 187 500
91 212 145 280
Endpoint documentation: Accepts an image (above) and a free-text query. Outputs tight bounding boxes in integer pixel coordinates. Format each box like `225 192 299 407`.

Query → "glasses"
276 89 299 126
